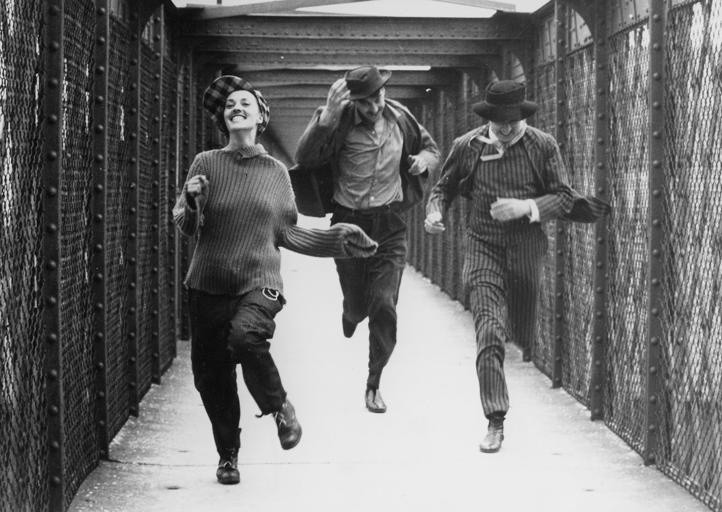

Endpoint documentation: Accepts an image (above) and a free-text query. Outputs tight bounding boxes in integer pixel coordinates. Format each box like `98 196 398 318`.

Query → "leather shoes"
216 452 241 485
342 315 357 338
364 386 388 414
273 399 303 451
479 417 505 453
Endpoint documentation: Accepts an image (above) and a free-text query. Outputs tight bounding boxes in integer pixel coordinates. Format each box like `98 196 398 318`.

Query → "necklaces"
367 123 377 134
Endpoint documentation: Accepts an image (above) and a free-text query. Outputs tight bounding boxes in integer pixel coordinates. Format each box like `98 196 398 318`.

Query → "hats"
202 74 270 139
472 79 540 123
340 64 393 101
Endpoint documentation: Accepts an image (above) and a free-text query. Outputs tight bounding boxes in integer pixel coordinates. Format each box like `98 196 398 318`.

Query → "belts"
336 198 400 218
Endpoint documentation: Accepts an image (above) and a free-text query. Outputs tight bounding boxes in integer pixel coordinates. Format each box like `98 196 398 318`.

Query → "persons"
296 64 441 412
172 74 379 484
424 80 612 454
